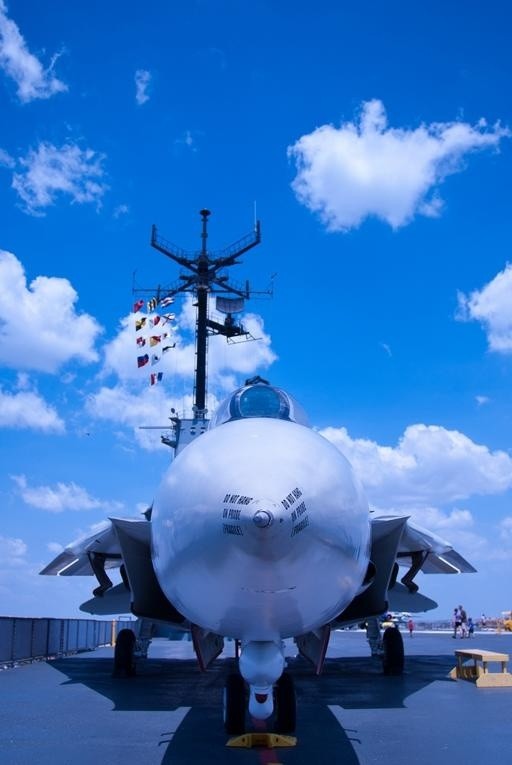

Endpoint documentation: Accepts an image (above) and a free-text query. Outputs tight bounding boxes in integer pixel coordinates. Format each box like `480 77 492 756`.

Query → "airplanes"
31 378 480 763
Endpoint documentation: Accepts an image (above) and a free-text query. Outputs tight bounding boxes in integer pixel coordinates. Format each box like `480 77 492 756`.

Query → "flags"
133 297 175 385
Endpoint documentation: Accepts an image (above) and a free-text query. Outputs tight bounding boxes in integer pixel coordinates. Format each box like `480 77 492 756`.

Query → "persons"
466 618 475 638
480 614 488 631
459 605 468 638
379 613 414 636
452 609 463 639
